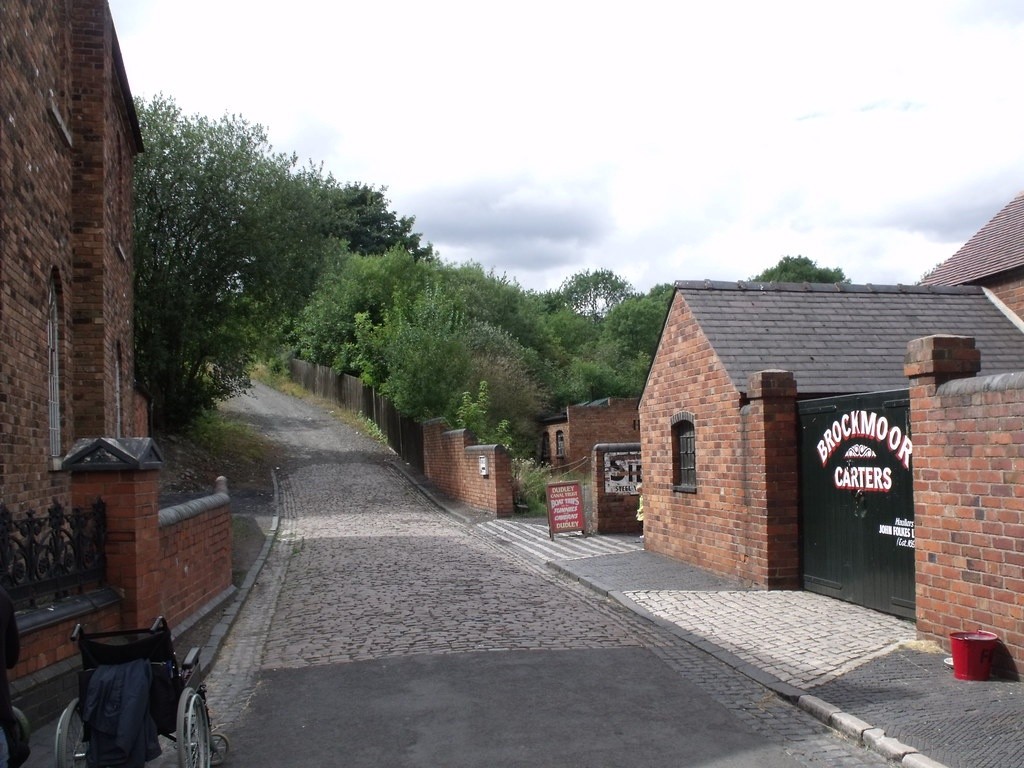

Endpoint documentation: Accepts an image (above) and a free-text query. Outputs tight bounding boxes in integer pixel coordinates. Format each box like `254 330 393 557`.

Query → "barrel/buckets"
950 631 998 681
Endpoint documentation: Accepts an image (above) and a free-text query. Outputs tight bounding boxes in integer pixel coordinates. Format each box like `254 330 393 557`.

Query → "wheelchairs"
54 616 230 768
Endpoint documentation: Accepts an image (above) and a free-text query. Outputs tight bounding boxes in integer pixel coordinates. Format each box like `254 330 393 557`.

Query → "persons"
635 484 645 540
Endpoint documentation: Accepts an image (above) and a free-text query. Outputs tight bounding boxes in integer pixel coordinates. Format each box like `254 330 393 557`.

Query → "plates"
944 657 954 668
